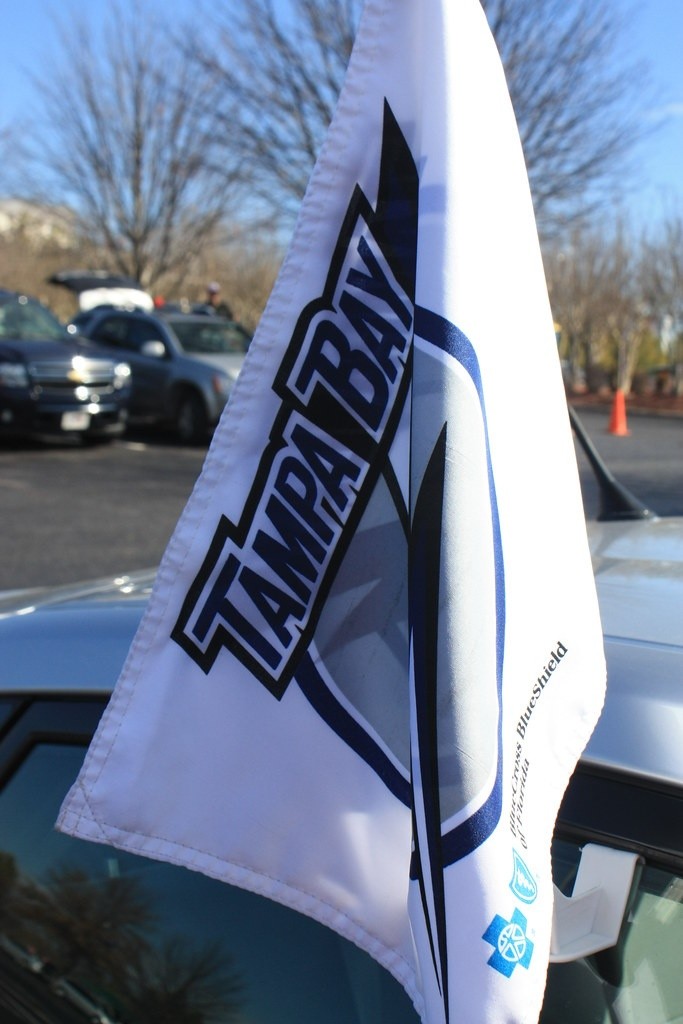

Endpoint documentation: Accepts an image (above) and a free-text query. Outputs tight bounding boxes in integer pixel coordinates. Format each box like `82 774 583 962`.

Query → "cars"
0 516 683 1024
0 285 132 444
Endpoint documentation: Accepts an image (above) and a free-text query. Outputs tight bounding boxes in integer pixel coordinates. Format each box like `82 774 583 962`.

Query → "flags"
59 0 607 1024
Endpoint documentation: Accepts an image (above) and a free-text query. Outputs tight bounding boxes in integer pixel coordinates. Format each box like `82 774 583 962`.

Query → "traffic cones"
609 389 629 436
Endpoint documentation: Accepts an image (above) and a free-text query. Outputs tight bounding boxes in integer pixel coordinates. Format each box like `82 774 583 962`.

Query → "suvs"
62 303 255 447
